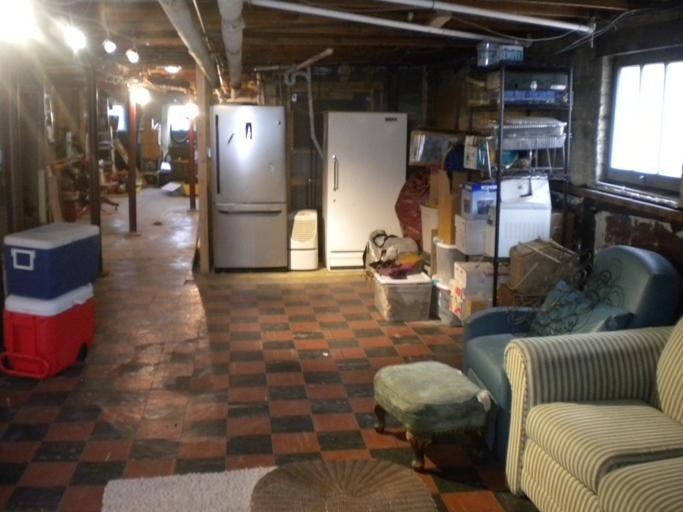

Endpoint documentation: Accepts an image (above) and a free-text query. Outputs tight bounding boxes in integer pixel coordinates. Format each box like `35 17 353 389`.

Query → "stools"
374 361 492 472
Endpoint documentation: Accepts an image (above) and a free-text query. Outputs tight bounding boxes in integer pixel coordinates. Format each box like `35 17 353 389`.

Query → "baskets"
506 235 581 298
496 282 544 308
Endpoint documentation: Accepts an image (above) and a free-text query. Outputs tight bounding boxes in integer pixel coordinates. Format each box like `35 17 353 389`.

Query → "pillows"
532 280 634 336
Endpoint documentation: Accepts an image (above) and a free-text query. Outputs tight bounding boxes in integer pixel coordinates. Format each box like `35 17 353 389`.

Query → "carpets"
102 465 278 512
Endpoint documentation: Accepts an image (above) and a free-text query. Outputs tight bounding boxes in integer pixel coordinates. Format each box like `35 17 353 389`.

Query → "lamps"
30 8 148 65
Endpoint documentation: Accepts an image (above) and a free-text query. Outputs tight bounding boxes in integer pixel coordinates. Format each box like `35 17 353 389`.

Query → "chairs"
464 245 683 462
501 316 683 512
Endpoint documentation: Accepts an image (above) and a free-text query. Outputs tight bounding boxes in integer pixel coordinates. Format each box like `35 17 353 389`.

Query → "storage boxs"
375 176 552 327
2 283 94 381
4 223 101 299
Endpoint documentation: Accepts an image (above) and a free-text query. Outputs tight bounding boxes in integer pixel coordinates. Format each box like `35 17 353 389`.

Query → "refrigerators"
324 110 409 269
211 103 288 270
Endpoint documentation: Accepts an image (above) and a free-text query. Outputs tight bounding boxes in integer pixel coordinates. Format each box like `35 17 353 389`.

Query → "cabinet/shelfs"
457 61 574 246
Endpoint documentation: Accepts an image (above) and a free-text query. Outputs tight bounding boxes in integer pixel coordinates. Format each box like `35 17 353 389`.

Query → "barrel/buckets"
419 203 440 255
